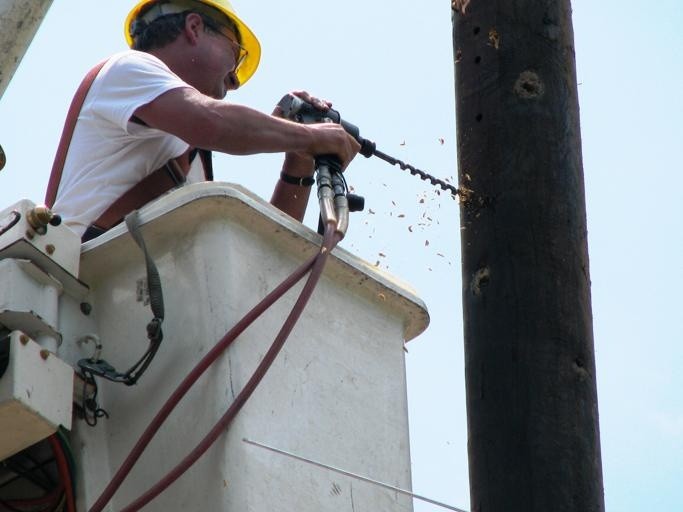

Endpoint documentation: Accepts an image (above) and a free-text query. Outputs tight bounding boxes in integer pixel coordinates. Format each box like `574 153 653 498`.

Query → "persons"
46 1 362 242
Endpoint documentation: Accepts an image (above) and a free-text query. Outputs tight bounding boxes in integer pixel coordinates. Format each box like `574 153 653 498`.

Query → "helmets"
121 0 263 88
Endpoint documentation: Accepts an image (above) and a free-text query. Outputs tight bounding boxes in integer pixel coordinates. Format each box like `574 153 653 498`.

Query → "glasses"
200 18 250 78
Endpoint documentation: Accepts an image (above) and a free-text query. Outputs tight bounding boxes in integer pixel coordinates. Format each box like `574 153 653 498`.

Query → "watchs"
278 171 316 187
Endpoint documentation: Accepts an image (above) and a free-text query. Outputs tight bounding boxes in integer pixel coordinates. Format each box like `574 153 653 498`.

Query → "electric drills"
279 93 472 220
